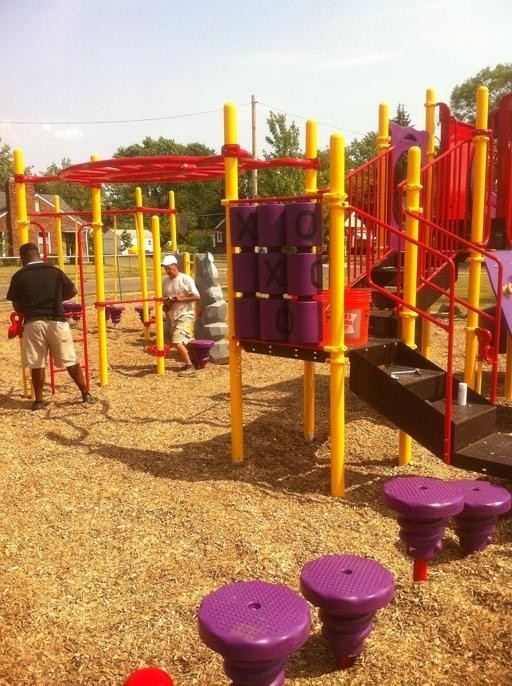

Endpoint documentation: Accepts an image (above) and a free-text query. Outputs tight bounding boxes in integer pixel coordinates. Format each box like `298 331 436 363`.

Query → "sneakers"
177 367 198 376
32 400 48 411
83 392 91 403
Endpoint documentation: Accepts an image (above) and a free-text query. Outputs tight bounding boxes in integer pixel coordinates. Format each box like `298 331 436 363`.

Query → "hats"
161 255 178 267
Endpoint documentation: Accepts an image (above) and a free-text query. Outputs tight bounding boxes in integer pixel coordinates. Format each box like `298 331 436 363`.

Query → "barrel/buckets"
290 295 330 346
322 289 372 347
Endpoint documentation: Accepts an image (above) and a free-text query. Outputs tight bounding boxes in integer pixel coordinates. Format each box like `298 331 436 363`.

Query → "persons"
160 255 201 376
7 242 92 410
